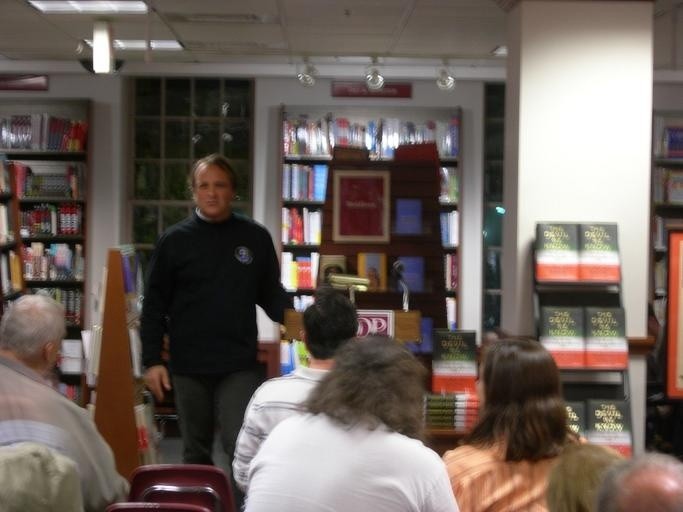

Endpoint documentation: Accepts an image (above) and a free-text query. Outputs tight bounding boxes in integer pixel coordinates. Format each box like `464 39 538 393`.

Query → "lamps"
364 54 385 89
433 56 454 89
296 57 314 85
92 20 112 74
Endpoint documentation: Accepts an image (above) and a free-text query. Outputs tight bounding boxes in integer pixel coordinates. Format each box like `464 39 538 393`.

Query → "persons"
0 290 132 512
596 446 683 512
543 440 625 511
442 336 590 512
138 149 304 512
231 282 361 496
239 330 463 512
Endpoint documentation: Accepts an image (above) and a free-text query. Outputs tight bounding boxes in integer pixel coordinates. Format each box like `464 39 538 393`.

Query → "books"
0 112 89 404
281 109 482 434
646 110 683 385
535 221 631 460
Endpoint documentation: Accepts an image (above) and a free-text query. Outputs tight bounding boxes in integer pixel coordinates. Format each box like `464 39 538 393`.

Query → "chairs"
108 464 235 511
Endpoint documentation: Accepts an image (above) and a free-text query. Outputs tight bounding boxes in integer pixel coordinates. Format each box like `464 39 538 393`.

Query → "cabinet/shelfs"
531 240 633 456
652 108 683 370
92 242 154 479
0 90 92 350
277 102 462 331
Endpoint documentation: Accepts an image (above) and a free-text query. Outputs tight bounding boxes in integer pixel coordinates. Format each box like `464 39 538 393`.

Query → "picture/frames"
332 167 391 243
